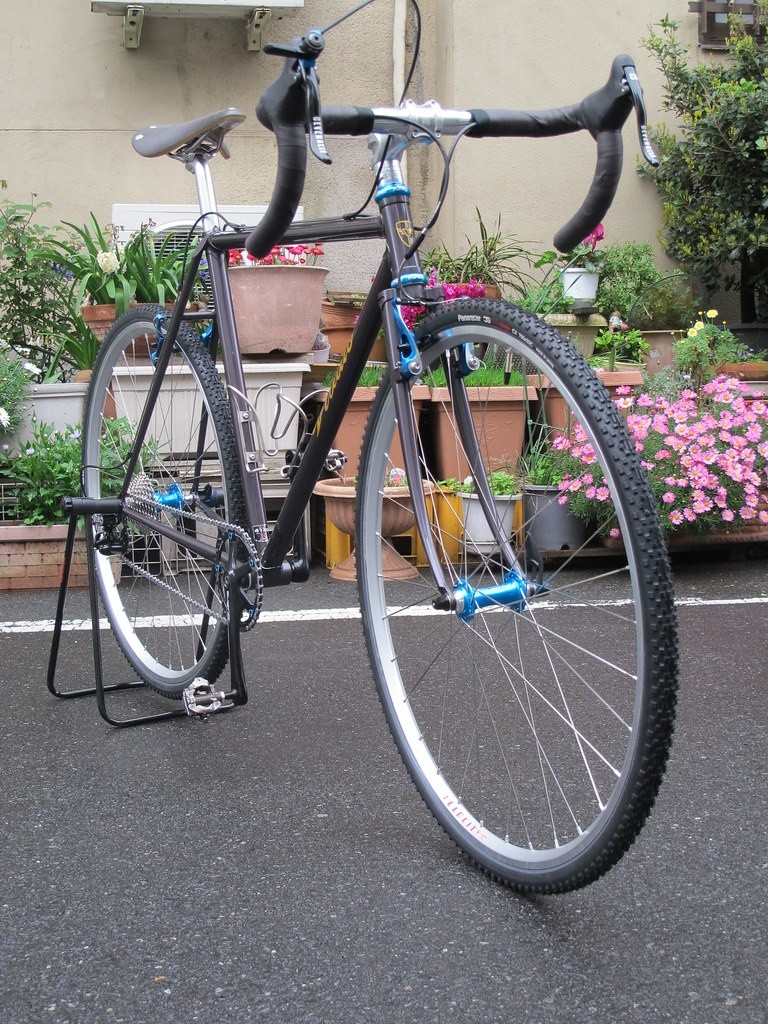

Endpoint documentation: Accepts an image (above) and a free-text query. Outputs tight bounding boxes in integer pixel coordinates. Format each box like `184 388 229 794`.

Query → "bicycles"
46 0 682 897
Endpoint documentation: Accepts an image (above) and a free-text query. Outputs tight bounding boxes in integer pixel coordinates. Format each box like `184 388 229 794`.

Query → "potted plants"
494 353 594 554
0 190 94 460
309 362 431 478
416 205 549 302
30 278 118 447
311 471 434 582
437 471 522 555
422 363 539 483
523 347 645 451
701 362 768 381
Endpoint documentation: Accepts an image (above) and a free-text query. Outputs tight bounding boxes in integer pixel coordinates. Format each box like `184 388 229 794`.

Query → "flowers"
534 220 608 273
227 241 327 267
35 217 207 321
547 308 768 547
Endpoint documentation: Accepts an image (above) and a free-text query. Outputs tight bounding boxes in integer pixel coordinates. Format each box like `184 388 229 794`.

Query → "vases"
227 265 330 355
558 267 602 303
78 302 199 356
